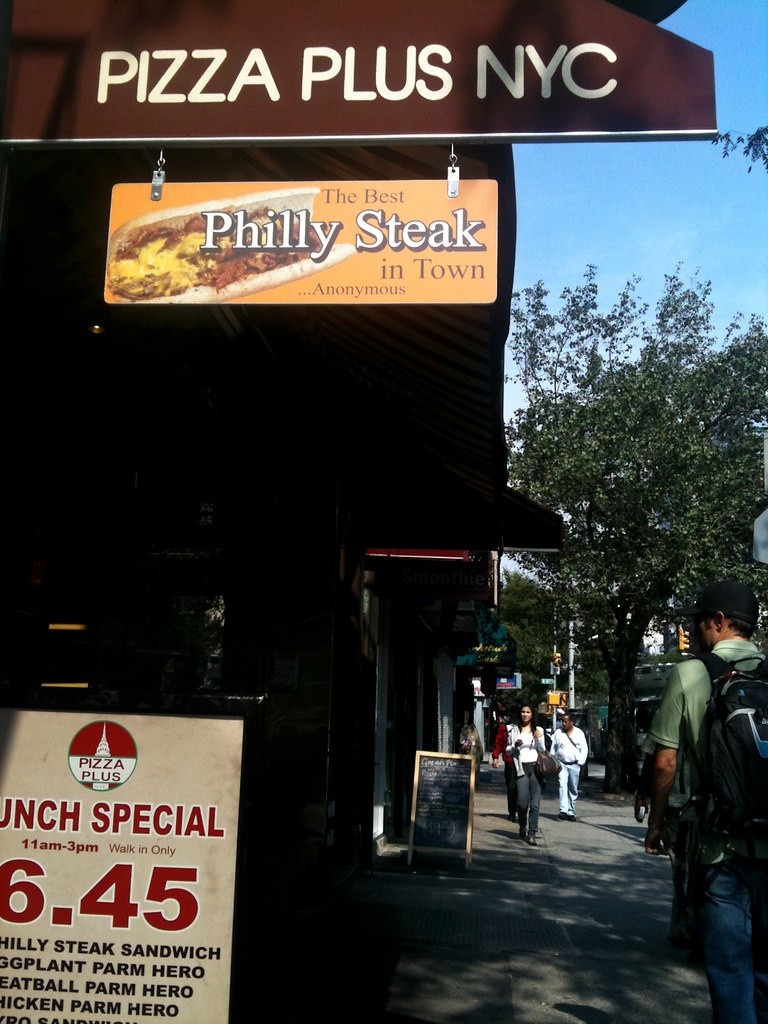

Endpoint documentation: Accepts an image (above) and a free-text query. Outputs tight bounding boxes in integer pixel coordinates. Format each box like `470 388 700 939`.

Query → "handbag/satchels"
536 748 561 777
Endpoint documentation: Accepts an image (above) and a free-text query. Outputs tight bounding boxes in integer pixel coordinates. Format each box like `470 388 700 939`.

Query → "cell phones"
518 740 522 742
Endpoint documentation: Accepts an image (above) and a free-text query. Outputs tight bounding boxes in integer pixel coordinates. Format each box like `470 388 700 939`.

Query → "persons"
504 703 547 846
548 714 589 822
645 580 768 1024
634 708 688 949
491 707 521 822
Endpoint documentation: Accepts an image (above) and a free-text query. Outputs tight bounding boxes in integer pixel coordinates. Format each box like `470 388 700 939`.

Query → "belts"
564 762 576 764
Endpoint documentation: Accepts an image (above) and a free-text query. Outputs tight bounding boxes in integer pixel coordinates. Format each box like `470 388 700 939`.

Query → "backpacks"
689 653 768 841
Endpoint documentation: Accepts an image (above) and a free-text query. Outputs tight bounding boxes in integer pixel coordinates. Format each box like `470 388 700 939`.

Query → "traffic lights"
559 691 568 708
678 626 689 651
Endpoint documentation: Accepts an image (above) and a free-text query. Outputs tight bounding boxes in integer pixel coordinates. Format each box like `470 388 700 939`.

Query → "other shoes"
568 816 576 821
512 814 518 821
528 838 536 845
559 812 566 819
519 826 526 837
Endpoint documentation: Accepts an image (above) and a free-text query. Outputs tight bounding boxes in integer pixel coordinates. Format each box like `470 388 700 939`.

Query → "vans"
634 696 664 775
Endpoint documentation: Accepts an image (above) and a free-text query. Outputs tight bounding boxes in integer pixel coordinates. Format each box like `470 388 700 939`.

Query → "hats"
676 582 759 625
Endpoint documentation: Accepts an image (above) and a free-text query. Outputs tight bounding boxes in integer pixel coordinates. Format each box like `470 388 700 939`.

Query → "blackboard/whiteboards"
409 750 476 855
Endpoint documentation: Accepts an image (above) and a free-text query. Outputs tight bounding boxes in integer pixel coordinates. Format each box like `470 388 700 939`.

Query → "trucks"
587 706 606 764
